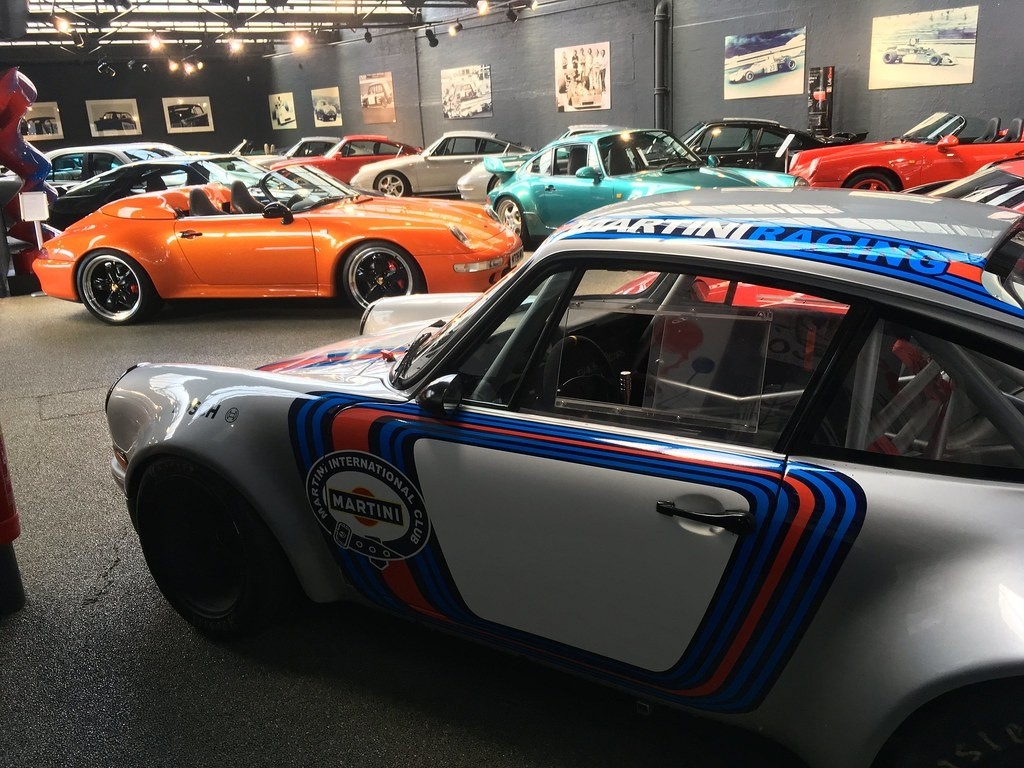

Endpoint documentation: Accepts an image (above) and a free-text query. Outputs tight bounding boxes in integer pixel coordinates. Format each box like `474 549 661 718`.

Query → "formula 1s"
272 104 295 126
728 55 796 82
882 44 959 66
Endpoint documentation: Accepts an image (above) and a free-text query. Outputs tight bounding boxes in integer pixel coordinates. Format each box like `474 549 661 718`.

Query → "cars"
315 100 337 121
0 142 187 205
362 82 392 108
632 117 869 172
93 111 137 131
168 104 209 128
484 128 810 248
0 425 26 610
457 125 634 205
349 130 539 198
105 187 1024 768
610 160 1024 318
19 117 58 136
787 112 1024 191
227 136 373 172
270 135 425 185
47 154 395 233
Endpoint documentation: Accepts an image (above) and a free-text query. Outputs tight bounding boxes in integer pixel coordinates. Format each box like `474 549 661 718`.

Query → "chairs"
146 175 167 192
230 179 265 215
373 142 381 154
567 148 587 175
608 147 632 176
737 132 754 151
974 116 1001 143
475 138 487 153
190 188 229 215
996 118 1024 142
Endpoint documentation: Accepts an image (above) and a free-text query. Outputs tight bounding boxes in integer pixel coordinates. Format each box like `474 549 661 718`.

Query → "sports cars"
31 164 524 326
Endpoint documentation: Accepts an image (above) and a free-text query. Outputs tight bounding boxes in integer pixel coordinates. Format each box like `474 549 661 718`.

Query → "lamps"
150 29 161 48
96 49 116 77
507 3 518 23
127 58 137 70
194 58 203 70
425 26 439 48
448 18 464 36
141 61 152 74
364 27 372 43
48 2 69 31
72 17 85 47
